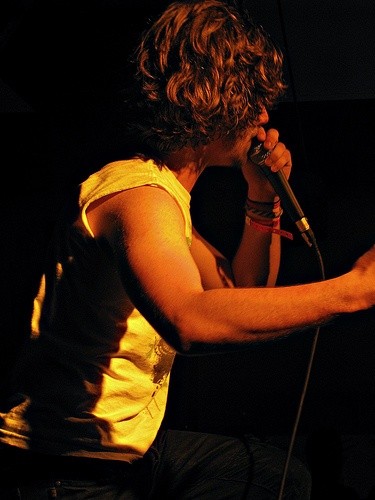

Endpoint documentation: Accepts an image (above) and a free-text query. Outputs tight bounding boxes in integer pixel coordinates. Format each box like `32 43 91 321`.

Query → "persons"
24 0 374 499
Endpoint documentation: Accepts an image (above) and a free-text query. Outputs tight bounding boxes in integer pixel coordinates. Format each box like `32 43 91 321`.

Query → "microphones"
246 141 317 250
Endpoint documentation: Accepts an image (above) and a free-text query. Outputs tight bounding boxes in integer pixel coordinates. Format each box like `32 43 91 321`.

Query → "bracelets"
244 198 293 240
